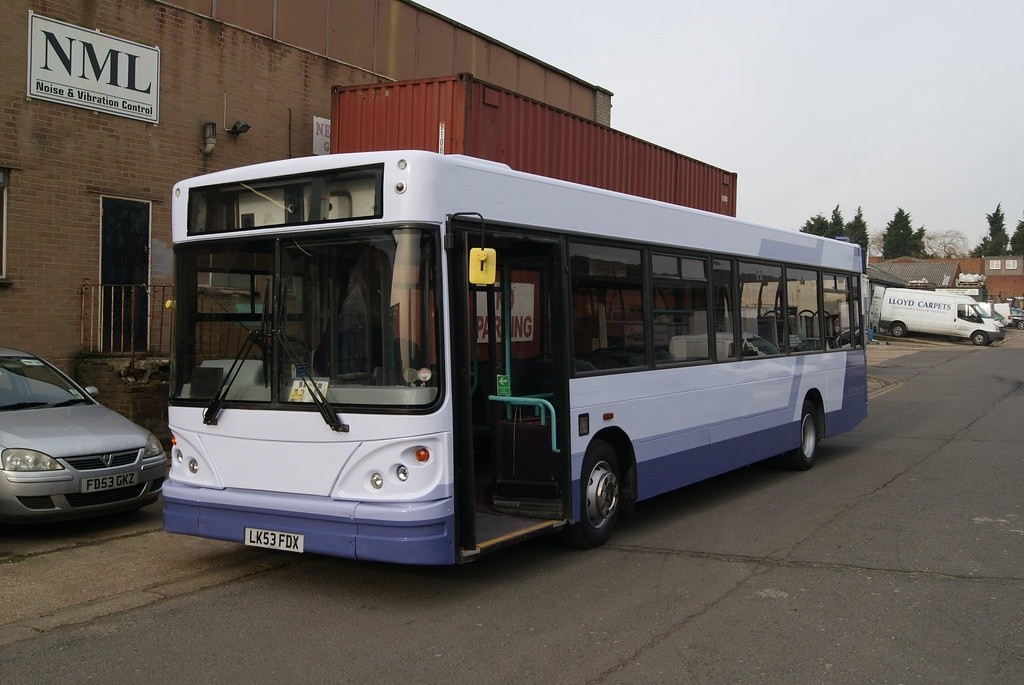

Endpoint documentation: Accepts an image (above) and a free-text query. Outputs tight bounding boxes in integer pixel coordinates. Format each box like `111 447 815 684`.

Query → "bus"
161 150 868 566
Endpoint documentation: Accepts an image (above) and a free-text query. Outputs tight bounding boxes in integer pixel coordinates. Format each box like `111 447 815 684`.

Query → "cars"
1009 307 1024 330
0 345 169 526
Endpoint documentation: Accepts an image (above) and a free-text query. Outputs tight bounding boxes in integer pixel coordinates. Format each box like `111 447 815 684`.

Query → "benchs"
536 338 840 382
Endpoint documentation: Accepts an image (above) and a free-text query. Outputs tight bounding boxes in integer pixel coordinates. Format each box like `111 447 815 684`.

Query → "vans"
880 286 1006 346
